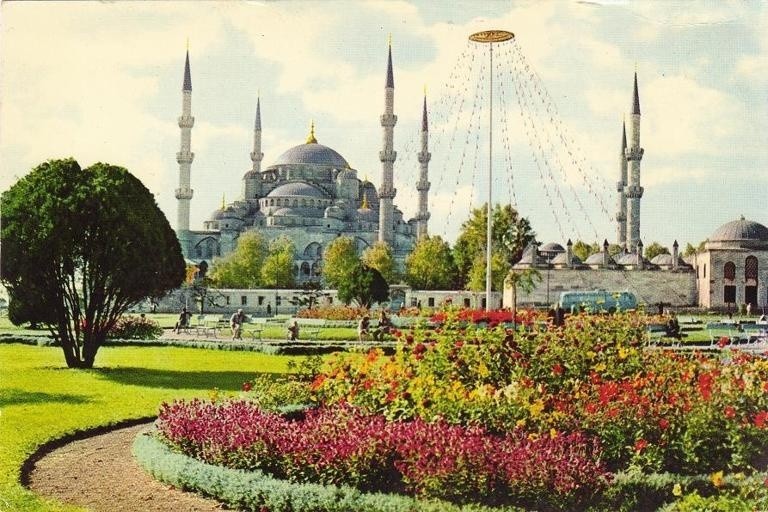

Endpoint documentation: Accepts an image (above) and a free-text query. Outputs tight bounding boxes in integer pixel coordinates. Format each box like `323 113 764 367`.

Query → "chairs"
179 313 189 334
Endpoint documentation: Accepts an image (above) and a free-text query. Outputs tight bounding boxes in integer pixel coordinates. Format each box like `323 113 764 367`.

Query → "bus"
558 290 637 314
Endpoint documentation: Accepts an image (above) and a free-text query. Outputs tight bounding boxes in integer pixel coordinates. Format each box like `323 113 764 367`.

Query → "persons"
288 321 298 341
358 315 369 344
663 320 682 341
372 311 392 341
230 309 244 341
670 314 680 333
547 301 565 327
658 302 663 317
747 303 752 317
172 308 193 334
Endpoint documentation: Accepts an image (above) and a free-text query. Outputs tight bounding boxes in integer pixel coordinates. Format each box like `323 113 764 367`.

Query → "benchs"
646 323 684 348
741 325 768 344
248 329 263 341
196 320 218 339
677 316 704 334
705 323 741 346
216 319 231 330
